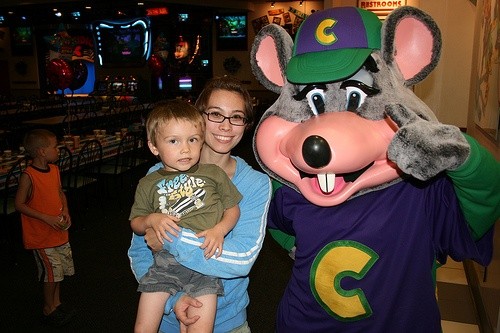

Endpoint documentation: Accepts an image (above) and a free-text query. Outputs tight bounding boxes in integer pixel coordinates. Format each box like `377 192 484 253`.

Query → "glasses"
202 109 248 126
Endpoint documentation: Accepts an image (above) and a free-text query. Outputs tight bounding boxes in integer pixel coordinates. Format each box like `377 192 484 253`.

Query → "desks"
23 103 157 142
0 130 134 196
0 99 115 143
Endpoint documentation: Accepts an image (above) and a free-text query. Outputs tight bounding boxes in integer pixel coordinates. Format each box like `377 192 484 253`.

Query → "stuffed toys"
250 6 499 333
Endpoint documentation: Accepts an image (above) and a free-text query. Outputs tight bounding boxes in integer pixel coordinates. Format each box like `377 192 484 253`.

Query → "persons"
129 100 243 333
128 77 273 332
14 130 74 319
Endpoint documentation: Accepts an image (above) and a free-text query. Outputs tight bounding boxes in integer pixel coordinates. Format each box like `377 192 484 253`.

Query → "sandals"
56 303 77 315
40 309 72 324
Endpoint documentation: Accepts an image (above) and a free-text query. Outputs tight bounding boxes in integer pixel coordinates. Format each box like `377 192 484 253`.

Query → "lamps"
270 2 275 7
300 1 303 7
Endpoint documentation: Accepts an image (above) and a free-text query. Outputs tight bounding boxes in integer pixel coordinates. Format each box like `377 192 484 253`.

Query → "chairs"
0 88 173 275
